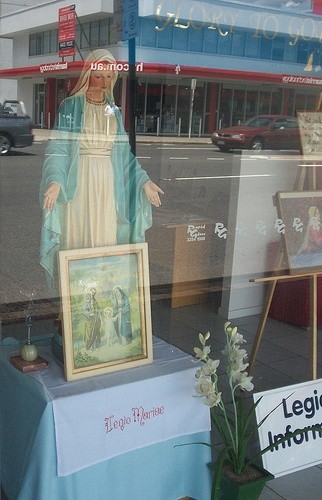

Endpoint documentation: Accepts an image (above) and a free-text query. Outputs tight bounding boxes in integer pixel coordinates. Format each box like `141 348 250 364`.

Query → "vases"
207 455 275 500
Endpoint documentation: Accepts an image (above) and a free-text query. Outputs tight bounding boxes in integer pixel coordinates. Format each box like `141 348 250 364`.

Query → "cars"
210 114 319 155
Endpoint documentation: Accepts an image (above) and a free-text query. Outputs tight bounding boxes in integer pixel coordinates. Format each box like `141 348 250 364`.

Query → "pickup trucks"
0 99 33 154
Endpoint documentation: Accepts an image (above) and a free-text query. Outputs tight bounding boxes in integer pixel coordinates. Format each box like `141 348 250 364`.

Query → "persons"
297 206 322 255
40 49 163 367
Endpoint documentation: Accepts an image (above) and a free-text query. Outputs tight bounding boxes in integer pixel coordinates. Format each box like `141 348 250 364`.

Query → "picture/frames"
59 242 153 381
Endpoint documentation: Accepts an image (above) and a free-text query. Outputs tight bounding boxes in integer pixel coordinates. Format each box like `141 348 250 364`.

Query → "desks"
144 211 223 309
0 335 216 500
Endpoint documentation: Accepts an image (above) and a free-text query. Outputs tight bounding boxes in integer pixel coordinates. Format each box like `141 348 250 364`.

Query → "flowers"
192 321 256 459
